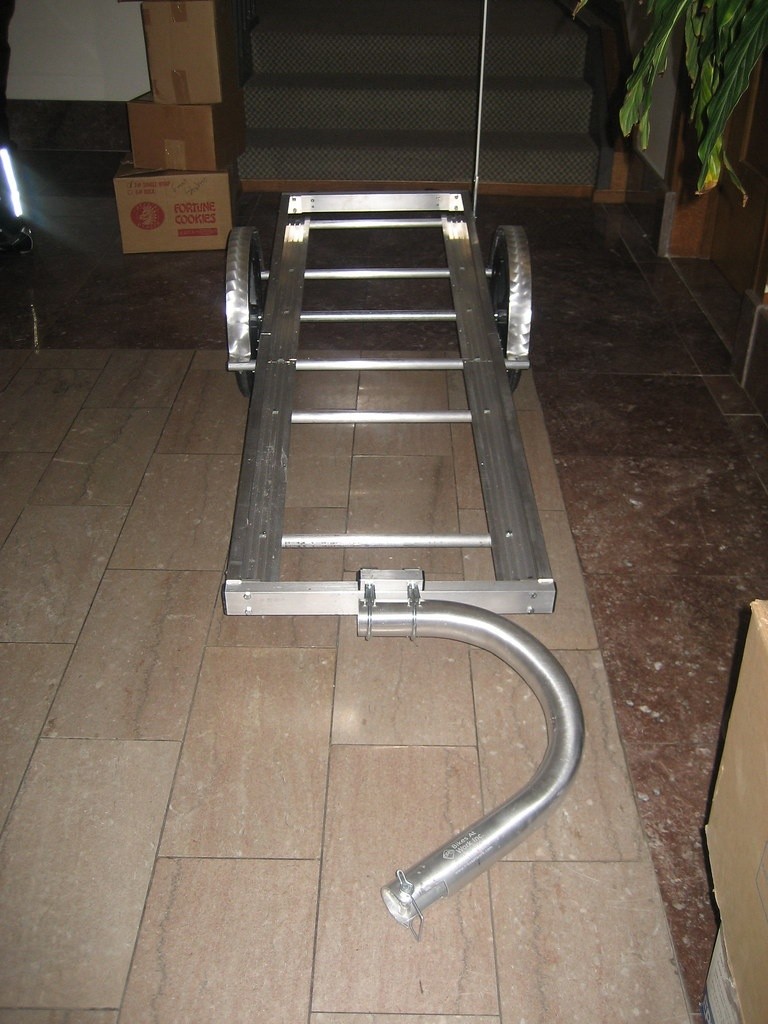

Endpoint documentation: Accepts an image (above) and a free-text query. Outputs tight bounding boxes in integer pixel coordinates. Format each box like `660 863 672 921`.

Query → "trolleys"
221 184 586 931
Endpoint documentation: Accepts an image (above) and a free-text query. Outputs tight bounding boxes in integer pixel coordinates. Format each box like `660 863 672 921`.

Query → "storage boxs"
701 922 744 1024
128 90 244 172
114 152 239 253
704 599 768 1024
140 0 239 104
